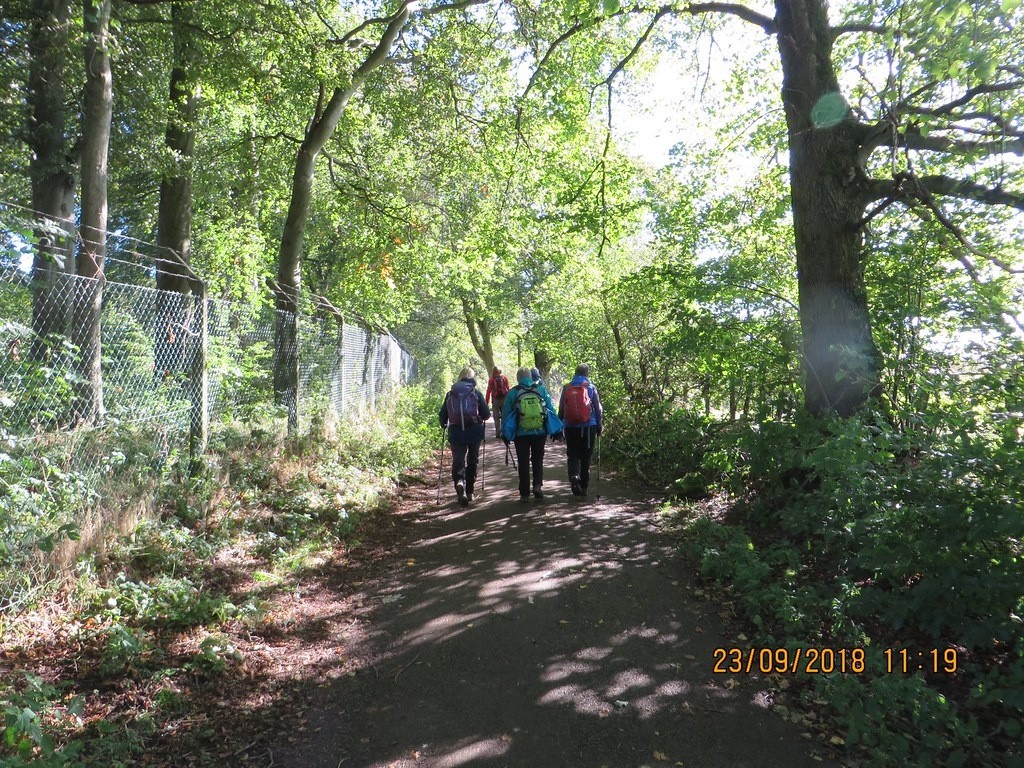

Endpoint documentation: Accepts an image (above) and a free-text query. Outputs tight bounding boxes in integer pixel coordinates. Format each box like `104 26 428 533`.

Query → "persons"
486 366 564 503
439 367 490 507
558 363 603 496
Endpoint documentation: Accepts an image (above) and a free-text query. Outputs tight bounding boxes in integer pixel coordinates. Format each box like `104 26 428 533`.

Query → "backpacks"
563 381 592 425
493 376 508 398
513 384 548 431
446 387 479 431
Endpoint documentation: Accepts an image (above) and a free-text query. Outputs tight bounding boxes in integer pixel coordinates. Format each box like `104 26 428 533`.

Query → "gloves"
597 423 604 437
501 434 510 445
550 431 566 442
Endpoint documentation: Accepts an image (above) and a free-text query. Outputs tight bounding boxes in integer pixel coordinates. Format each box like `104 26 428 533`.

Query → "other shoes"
520 494 529 502
571 479 588 496
467 493 474 501
496 435 500 438
456 485 468 506
532 485 543 498
